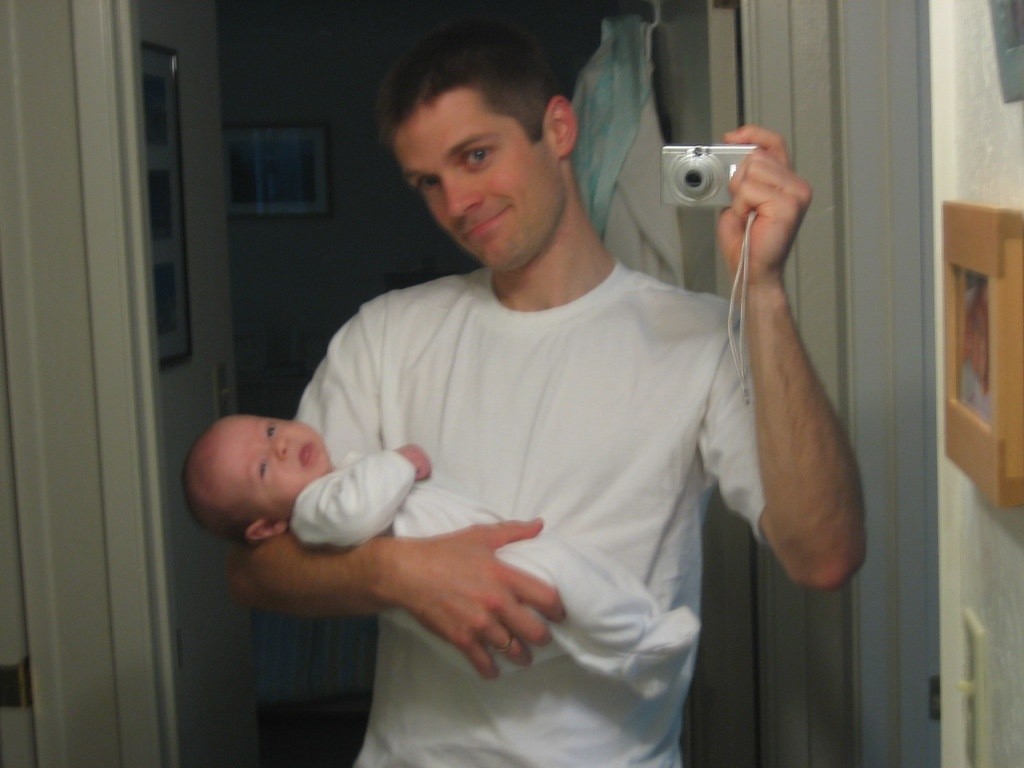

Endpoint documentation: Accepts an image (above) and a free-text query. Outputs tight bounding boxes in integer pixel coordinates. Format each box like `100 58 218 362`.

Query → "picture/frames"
963 607 988 768
221 121 335 222
943 202 1024 507
140 40 192 368
990 0 1024 102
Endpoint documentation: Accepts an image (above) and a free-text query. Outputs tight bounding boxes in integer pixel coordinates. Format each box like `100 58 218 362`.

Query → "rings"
496 635 513 652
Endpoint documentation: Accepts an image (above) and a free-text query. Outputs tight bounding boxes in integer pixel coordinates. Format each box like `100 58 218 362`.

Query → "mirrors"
0 0 941 768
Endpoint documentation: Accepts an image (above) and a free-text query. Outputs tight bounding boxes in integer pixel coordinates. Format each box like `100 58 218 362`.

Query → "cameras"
660 143 760 207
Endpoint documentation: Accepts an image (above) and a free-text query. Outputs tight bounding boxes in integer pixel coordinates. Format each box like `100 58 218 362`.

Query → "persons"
181 413 700 715
228 15 868 768
